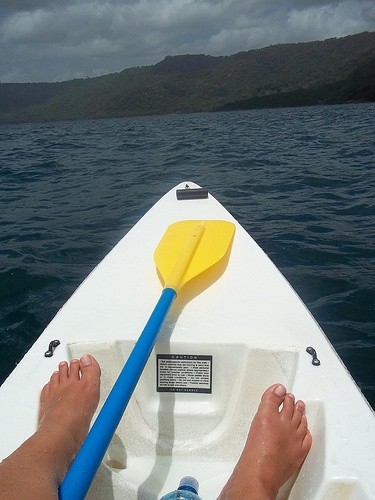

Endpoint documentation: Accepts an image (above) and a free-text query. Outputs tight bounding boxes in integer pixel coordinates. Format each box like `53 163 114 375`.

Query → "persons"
0 354 311 500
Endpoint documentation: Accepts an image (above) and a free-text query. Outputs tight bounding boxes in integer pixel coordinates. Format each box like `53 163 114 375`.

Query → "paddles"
56 219 237 500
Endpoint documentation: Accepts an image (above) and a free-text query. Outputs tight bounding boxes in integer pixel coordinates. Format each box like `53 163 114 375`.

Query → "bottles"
161 476 201 500
